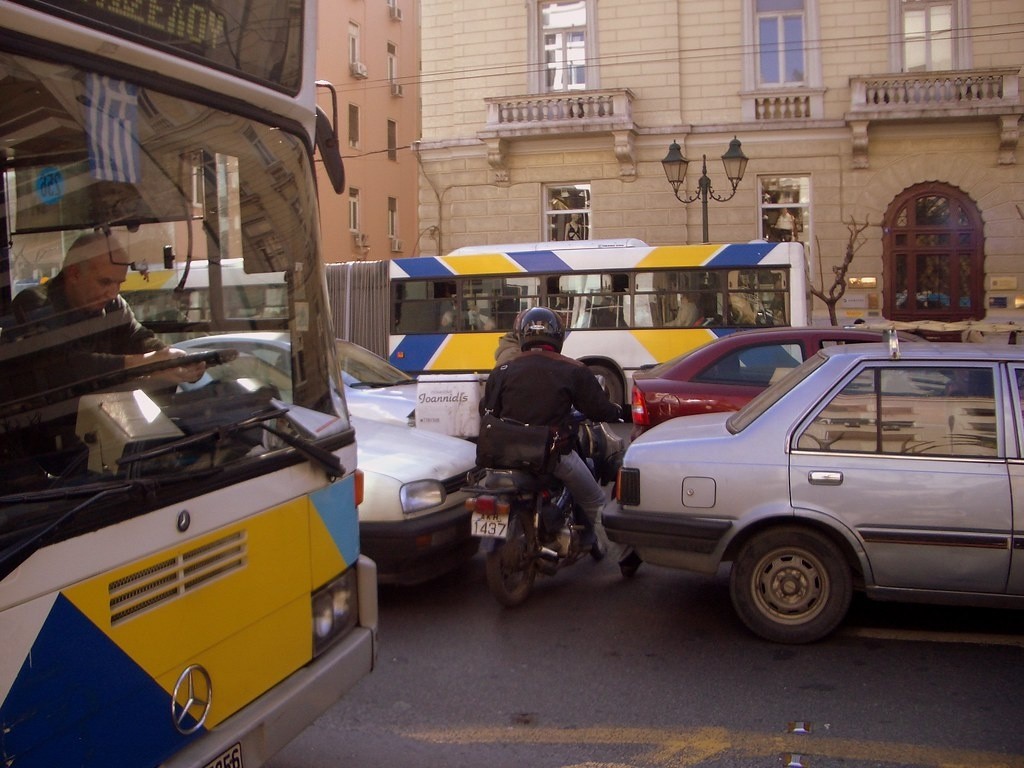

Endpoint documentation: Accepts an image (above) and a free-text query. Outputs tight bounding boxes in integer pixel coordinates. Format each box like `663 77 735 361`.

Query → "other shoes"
619 549 643 577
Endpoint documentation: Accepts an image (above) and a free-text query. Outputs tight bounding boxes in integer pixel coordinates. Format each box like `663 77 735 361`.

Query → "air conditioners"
392 84 404 97
352 61 367 78
390 7 403 21
392 239 405 253
355 232 369 246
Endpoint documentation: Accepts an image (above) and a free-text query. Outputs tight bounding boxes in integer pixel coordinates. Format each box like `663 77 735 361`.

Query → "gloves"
618 402 633 423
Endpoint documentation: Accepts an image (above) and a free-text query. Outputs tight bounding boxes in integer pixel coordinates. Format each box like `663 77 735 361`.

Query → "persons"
0 230 206 471
440 291 701 328
564 214 582 241
478 277 622 561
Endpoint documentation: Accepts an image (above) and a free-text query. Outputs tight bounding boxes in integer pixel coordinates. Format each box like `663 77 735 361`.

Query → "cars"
601 341 1024 645
282 401 478 564
630 326 930 444
169 330 419 418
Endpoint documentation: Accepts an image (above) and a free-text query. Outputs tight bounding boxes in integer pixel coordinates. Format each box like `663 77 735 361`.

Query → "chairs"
717 354 741 383
571 312 594 328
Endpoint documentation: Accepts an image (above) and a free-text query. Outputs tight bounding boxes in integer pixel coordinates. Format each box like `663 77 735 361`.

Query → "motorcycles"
460 403 624 607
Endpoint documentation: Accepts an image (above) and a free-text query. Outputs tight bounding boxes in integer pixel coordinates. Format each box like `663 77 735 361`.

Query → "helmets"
519 306 565 353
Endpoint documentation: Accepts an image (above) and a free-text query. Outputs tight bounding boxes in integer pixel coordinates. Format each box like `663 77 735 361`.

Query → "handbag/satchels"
474 412 557 478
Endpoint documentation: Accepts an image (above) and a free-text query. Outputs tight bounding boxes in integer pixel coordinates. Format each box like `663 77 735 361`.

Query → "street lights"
662 134 749 248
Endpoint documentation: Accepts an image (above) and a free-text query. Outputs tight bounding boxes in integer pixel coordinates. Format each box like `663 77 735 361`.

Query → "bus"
321 239 813 419
0 0 379 768
